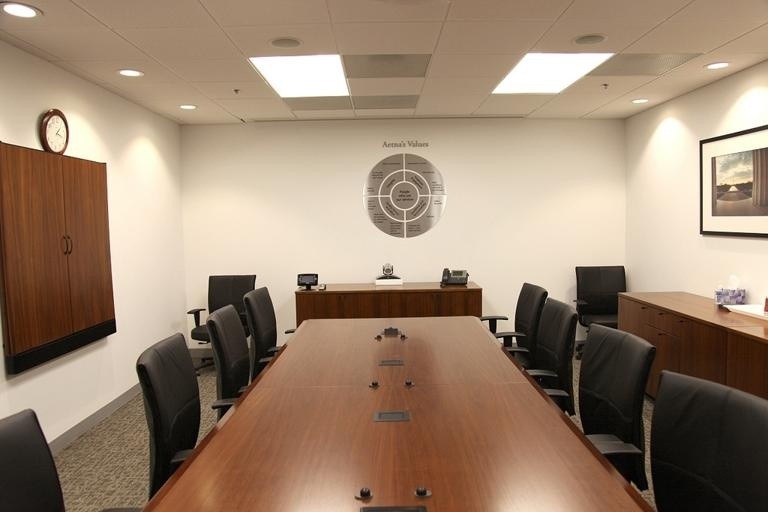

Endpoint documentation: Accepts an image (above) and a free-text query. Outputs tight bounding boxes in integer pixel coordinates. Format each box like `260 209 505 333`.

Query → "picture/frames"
699 125 768 237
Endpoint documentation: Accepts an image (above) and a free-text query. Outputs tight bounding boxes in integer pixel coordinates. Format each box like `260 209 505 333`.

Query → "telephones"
441 268 469 288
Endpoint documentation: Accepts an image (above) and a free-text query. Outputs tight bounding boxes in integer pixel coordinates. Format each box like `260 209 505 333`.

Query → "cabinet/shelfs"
0 141 146 379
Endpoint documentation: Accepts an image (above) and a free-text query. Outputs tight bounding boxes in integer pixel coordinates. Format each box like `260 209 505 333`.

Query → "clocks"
40 108 69 154
